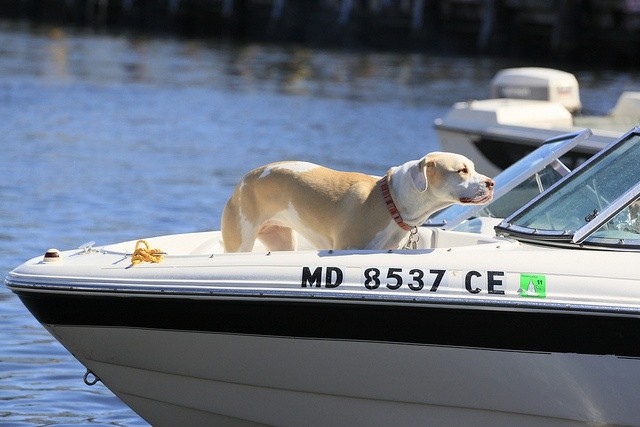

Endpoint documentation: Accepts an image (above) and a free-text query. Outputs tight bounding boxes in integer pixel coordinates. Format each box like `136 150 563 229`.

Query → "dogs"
221 152 495 252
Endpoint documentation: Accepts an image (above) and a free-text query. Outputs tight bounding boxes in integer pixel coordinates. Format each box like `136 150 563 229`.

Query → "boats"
434 68 640 218
4 126 640 427
